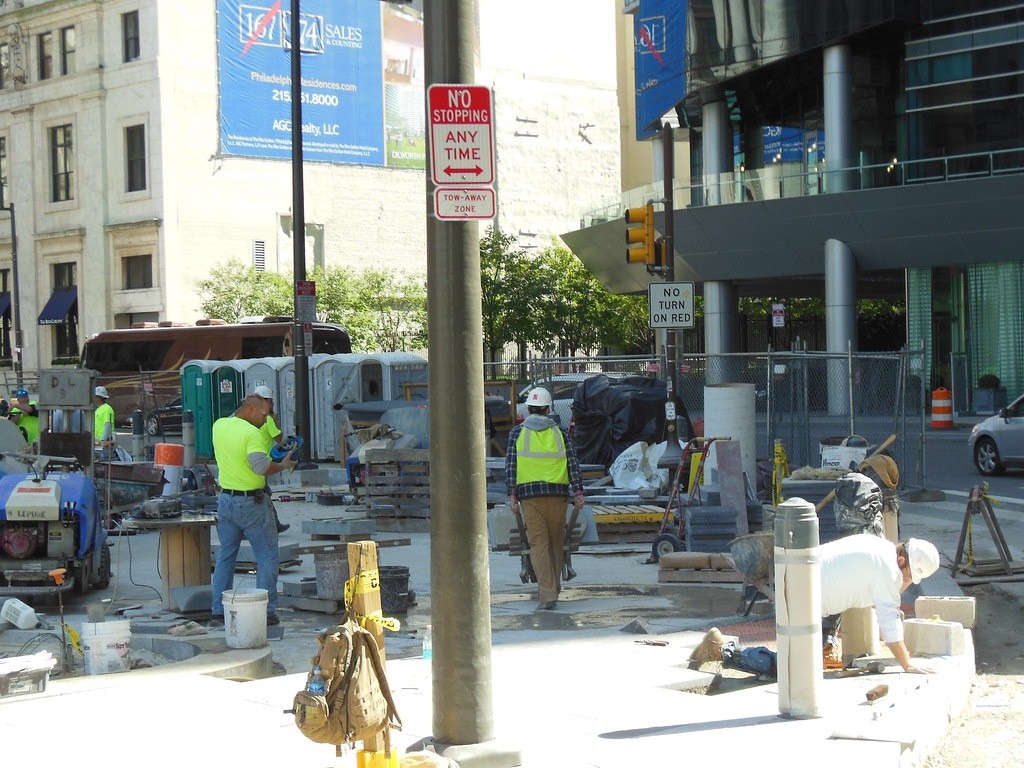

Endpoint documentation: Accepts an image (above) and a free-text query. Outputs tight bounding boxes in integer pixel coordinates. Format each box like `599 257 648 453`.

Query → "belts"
222 489 256 496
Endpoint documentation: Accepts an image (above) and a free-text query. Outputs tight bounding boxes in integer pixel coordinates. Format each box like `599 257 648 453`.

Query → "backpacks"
293 609 403 757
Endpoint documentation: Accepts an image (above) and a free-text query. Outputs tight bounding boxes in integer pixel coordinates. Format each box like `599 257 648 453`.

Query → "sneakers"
820 641 846 668
689 627 725 662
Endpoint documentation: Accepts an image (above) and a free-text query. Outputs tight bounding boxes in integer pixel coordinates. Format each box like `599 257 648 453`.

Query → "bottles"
423 624 433 660
0 598 42 630
309 665 325 696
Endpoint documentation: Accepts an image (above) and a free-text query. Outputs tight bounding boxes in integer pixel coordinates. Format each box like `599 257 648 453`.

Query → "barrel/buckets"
221 587 269 649
81 620 131 675
314 553 349 601
378 565 410 613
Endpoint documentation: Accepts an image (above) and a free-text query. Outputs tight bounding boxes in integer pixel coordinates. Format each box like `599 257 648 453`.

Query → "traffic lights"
626 204 655 264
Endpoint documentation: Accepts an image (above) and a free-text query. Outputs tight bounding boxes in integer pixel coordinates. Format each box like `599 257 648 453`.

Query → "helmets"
270 435 304 463
525 387 552 406
16 388 28 397
96 386 109 399
254 386 273 399
909 538 939 584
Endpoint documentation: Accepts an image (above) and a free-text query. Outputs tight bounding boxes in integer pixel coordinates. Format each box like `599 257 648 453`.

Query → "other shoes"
277 524 290 534
212 614 224 623
540 601 557 608
241 535 247 539
267 614 279 625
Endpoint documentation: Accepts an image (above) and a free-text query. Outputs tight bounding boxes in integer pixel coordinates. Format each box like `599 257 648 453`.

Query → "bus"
80 316 352 431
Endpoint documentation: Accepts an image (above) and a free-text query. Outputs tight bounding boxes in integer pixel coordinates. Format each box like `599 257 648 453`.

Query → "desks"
122 513 217 613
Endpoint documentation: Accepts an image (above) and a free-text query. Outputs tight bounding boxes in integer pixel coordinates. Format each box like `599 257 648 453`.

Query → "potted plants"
974 374 1007 414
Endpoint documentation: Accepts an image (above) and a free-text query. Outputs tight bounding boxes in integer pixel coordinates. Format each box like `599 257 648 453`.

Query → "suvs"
509 373 645 430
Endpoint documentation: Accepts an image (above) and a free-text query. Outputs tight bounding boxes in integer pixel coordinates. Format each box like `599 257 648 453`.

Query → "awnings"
39 285 78 325
0 291 11 319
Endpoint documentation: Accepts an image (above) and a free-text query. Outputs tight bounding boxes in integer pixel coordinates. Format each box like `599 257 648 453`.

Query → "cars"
968 392 1024 476
145 393 183 436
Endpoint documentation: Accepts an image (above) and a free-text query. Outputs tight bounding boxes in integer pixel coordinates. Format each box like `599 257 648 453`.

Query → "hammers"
836 661 885 679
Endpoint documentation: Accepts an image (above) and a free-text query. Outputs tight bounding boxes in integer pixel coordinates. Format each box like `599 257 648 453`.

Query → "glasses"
256 405 269 419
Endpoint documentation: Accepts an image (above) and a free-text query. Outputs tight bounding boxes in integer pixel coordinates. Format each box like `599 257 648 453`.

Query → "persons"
243 386 291 541
8 389 85 449
689 534 941 679
211 393 298 626
95 386 117 461
505 387 584 610
